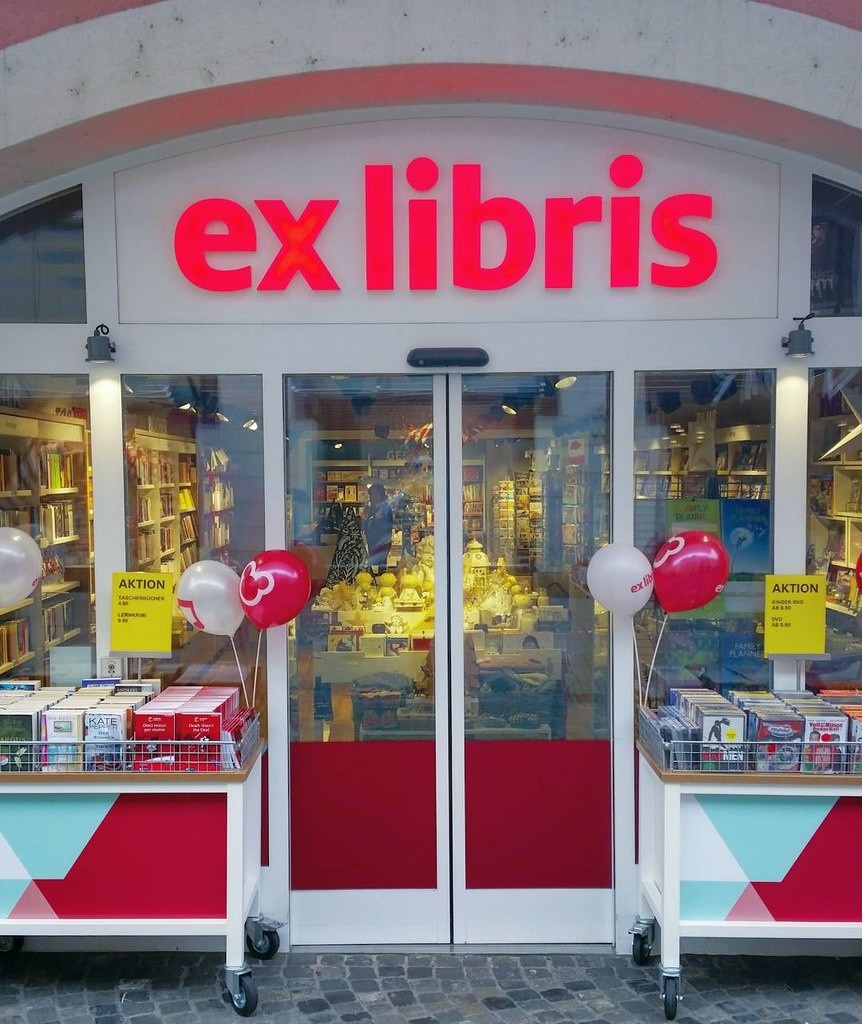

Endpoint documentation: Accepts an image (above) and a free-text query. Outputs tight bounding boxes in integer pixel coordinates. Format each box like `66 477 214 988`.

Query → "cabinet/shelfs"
562 411 862 618
1 409 92 678
299 428 486 571
491 466 547 573
125 427 237 651
628 679 862 1022
1 678 289 1018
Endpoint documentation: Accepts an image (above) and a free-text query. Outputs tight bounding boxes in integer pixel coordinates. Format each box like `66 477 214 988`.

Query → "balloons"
238 550 312 631
653 530 730 613
588 545 654 617
176 549 245 637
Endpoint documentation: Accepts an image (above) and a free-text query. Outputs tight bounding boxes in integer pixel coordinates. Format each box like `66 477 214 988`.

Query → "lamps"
499 391 524 417
780 312 821 358
544 372 577 391
85 323 118 367
170 386 262 431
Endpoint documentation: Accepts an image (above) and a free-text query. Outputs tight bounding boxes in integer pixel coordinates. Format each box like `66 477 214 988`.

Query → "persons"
522 636 542 650
416 535 434 581
360 484 392 572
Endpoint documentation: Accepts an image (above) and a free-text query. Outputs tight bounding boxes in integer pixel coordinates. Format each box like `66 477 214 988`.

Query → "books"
0 677 258 772
0 446 96 678
515 455 609 571
133 449 233 643
638 686 862 774
633 439 772 498
